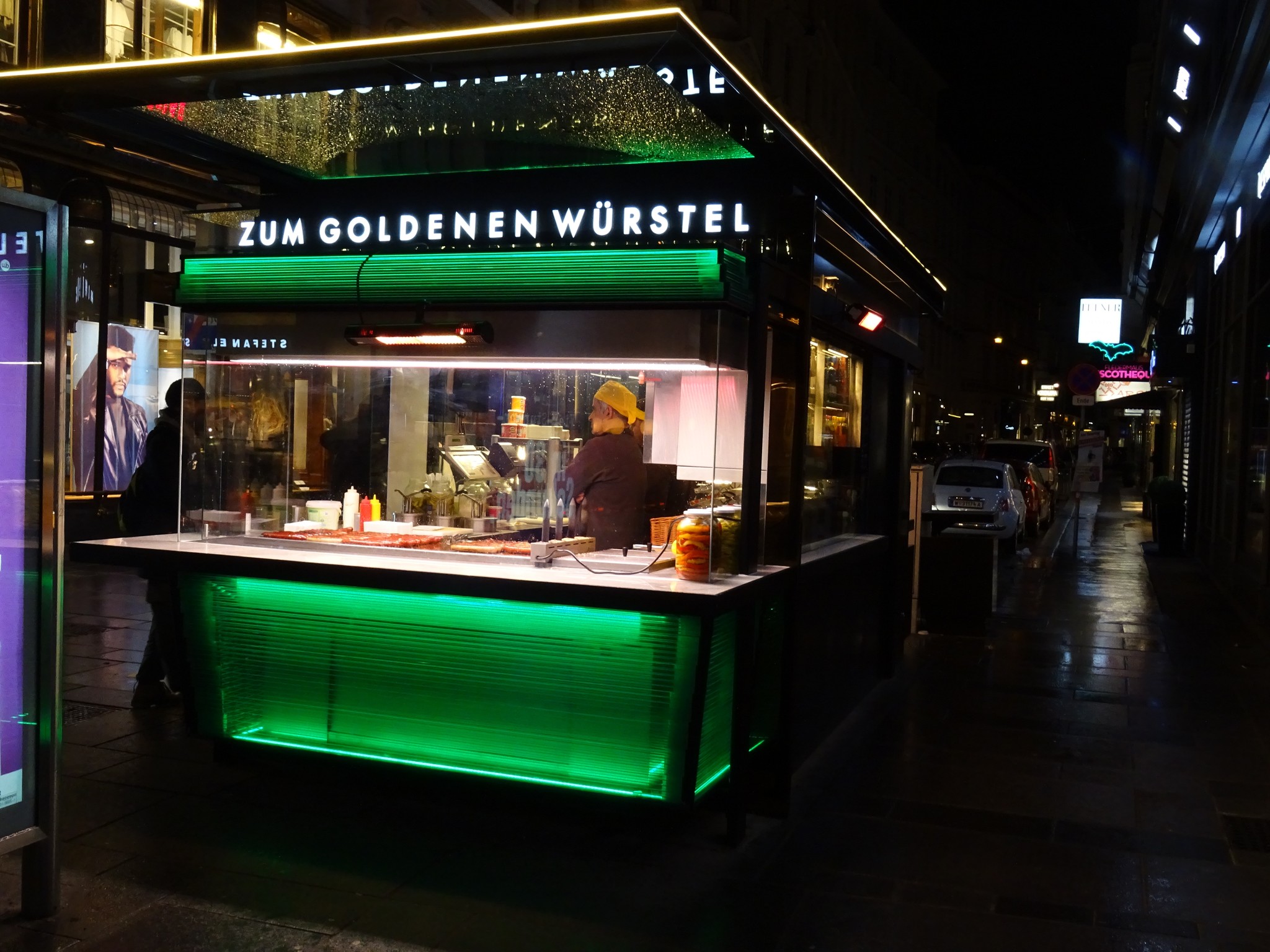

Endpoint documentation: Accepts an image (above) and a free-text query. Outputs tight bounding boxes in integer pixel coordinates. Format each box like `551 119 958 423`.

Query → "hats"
595 380 645 425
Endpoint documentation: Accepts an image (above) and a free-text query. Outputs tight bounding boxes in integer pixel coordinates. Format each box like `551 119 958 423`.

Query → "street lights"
1020 358 1037 430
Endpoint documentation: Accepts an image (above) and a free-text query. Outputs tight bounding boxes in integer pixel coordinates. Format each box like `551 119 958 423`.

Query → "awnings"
1095 390 1165 410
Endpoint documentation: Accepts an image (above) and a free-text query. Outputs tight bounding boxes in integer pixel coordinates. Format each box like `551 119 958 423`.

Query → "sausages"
450 538 531 555
261 527 443 548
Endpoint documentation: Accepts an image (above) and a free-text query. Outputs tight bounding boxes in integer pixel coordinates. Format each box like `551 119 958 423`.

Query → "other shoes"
131 679 183 711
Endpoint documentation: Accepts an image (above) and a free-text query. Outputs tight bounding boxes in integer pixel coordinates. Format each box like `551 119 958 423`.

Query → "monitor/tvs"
446 451 501 481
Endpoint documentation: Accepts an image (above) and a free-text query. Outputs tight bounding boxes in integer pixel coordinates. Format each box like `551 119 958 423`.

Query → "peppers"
675 524 721 581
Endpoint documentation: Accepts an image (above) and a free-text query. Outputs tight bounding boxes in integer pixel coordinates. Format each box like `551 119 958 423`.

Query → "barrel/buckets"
306 500 342 531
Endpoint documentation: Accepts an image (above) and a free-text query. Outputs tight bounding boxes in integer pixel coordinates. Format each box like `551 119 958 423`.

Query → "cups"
500 396 527 438
489 506 502 520
818 479 838 495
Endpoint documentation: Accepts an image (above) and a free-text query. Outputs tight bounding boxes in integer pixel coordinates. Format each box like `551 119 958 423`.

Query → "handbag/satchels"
119 465 146 536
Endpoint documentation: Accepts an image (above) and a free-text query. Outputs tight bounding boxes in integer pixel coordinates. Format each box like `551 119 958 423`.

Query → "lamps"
844 302 884 332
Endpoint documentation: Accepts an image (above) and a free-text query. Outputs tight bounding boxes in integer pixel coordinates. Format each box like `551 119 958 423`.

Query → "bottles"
808 346 848 404
825 414 849 448
239 482 286 530
342 486 380 532
675 504 742 583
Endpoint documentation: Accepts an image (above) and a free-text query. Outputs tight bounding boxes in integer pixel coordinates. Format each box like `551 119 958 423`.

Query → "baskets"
650 515 682 547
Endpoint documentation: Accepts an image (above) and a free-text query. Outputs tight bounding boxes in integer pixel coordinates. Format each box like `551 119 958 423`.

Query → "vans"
978 438 1114 524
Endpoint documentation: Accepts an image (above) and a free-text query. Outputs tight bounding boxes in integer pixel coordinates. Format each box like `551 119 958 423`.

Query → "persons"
131 376 221 709
554 379 704 552
72 323 149 492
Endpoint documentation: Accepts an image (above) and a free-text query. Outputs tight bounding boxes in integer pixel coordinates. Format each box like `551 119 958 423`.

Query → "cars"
988 457 1057 538
922 459 1033 556
